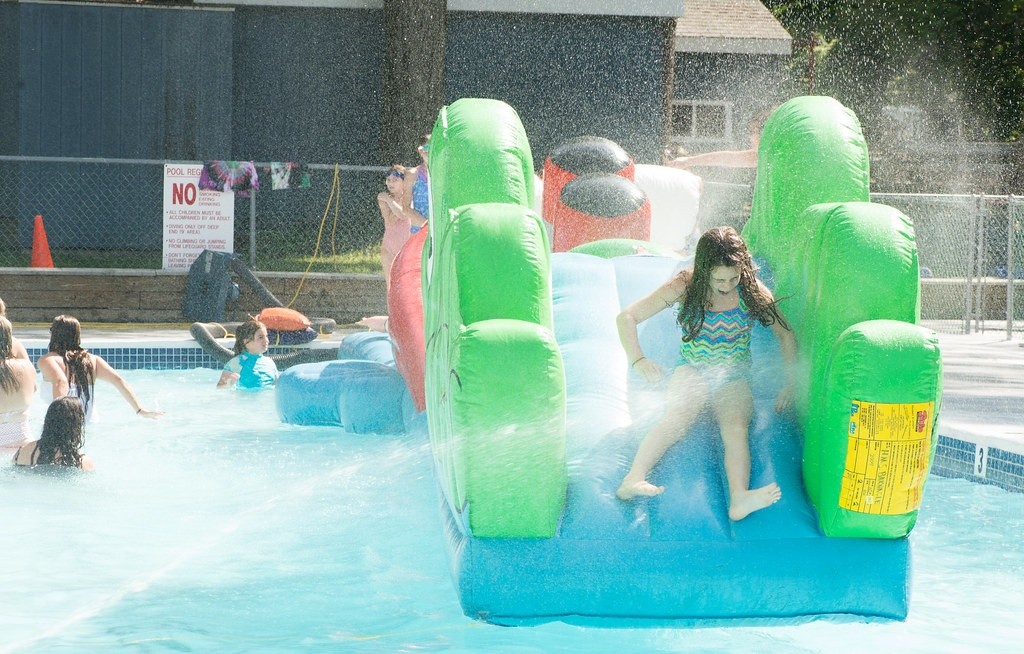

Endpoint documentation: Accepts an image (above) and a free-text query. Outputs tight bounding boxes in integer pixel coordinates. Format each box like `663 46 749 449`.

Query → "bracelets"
136 407 146 414
631 356 646 370
420 219 428 229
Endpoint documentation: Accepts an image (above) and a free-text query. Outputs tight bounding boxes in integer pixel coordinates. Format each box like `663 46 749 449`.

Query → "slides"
386 98 942 630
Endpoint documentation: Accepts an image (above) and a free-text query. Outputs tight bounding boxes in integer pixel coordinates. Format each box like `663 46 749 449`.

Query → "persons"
616 225 798 521
216 320 278 391
11 395 95 469
37 315 166 424
403 134 432 234
0 299 37 450
376 164 411 290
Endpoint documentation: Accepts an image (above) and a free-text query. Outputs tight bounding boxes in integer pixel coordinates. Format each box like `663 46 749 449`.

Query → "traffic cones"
30 214 56 269
